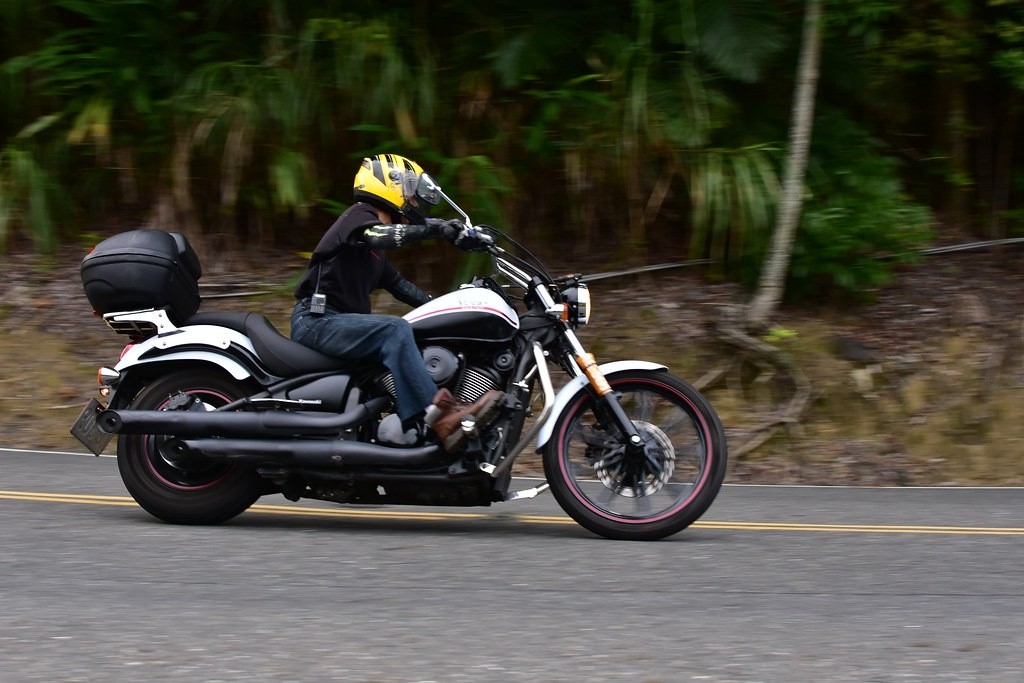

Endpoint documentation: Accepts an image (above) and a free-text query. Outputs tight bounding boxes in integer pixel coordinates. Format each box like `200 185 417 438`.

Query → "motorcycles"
67 171 730 543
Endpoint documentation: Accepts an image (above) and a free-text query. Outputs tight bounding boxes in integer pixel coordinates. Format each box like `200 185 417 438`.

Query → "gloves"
425 218 472 246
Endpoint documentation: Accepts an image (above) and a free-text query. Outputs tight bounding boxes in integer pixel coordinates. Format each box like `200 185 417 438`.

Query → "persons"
289 153 503 450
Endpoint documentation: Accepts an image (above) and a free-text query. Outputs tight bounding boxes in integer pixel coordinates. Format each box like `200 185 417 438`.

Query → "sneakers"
431 388 505 452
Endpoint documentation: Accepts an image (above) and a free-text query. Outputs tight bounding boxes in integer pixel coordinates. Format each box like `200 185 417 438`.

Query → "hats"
353 153 425 217
